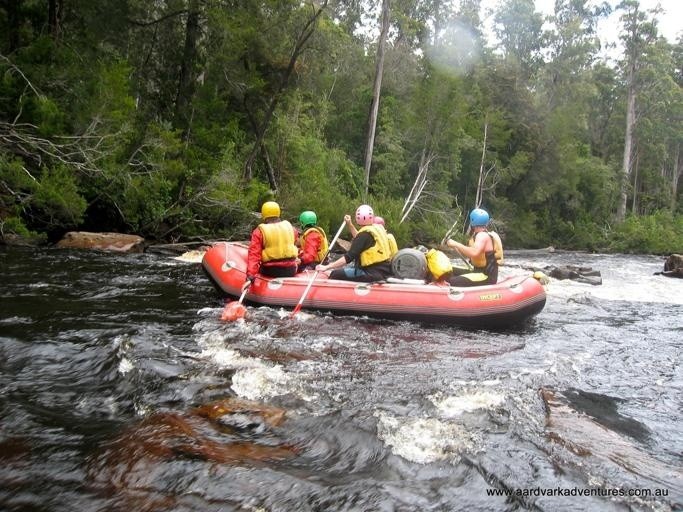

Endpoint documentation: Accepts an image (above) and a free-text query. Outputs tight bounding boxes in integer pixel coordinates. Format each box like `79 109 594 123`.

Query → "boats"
201 240 548 331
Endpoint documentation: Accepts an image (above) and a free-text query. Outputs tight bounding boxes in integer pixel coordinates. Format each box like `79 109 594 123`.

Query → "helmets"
356 205 373 226
470 209 489 226
300 211 317 228
262 202 280 218
375 217 384 225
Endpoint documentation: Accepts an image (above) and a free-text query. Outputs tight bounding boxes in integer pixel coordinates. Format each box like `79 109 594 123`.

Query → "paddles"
282 218 348 321
220 263 261 322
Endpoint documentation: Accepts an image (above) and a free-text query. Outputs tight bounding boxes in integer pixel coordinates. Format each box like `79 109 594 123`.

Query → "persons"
296 210 330 270
240 201 306 295
444 208 499 287
314 203 392 282
373 216 399 262
